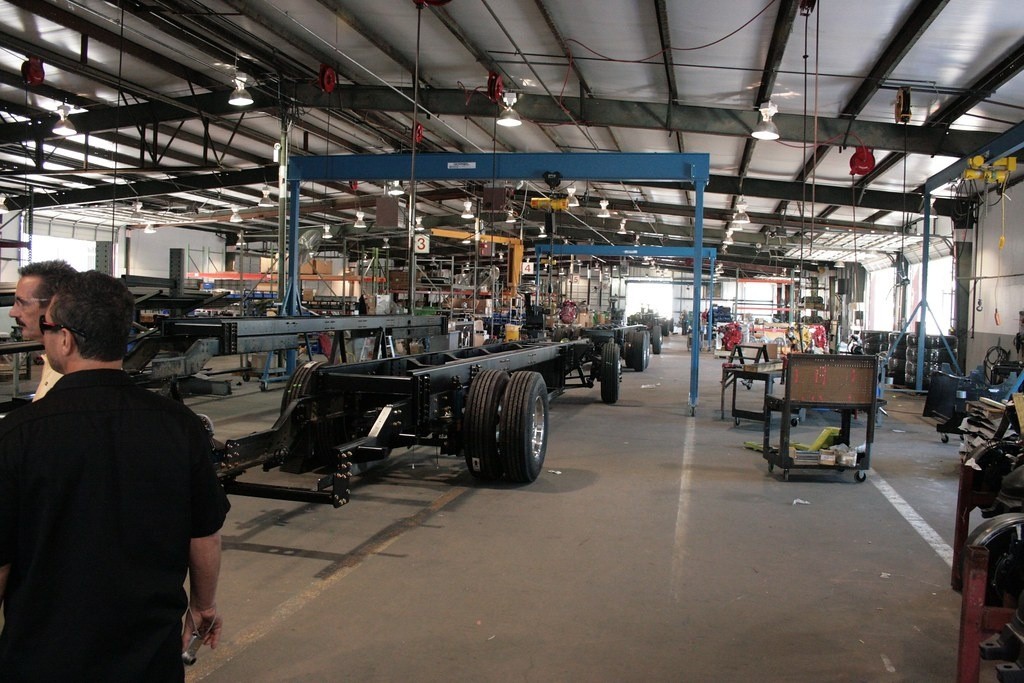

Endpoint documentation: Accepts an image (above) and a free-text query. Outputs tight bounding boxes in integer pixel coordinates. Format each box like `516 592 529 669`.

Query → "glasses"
39 315 92 341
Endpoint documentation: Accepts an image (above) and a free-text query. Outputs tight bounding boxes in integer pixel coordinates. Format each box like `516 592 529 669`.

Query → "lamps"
144 222 158 233
752 101 780 140
231 205 243 222
390 180 404 196
0 193 9 213
131 202 145 219
322 225 332 238
382 237 390 246
506 187 752 274
462 202 474 219
415 217 426 231
354 209 367 228
52 104 77 137
258 185 274 209
228 74 253 107
498 92 523 126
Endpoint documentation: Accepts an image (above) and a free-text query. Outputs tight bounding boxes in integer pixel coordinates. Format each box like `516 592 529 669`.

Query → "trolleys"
762 354 888 484
719 343 799 426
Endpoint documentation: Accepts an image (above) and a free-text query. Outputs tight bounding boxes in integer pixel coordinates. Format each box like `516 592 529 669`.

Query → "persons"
9 260 78 402
701 309 708 332
0 269 232 683
681 310 688 335
